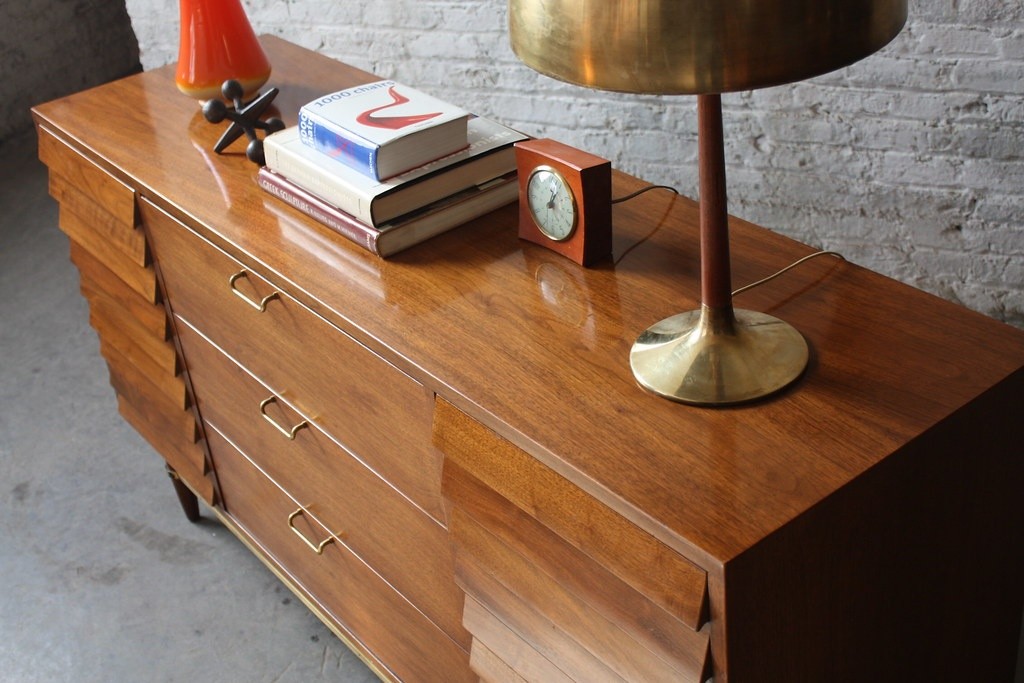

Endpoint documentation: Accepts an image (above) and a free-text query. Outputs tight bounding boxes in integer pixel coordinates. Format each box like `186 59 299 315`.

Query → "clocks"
514 138 612 266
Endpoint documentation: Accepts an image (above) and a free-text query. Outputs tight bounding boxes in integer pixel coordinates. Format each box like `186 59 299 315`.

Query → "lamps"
507 0 908 406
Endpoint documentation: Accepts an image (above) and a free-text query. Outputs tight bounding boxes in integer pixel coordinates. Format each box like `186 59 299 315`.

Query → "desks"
29 36 1024 683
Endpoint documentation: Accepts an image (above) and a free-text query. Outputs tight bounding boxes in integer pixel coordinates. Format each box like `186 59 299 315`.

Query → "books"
256 79 530 259
